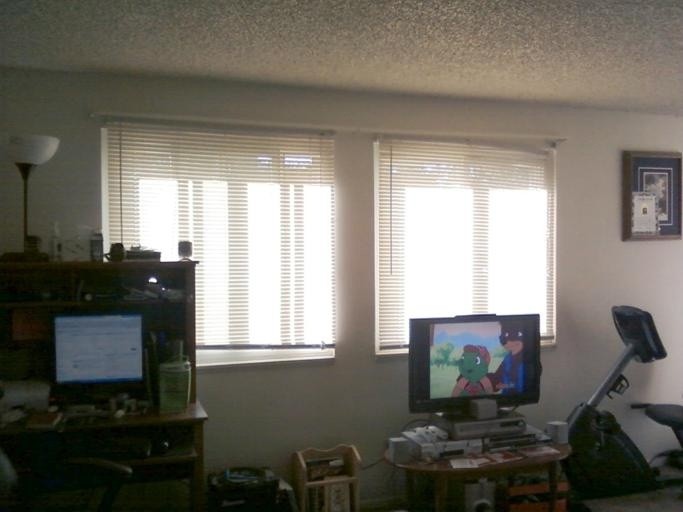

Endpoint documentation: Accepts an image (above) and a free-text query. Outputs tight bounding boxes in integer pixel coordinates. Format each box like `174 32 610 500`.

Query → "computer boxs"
430 413 526 440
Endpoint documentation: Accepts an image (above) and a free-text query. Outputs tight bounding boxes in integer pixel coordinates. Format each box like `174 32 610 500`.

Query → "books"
303 453 350 511
474 449 523 467
521 442 559 458
23 412 63 430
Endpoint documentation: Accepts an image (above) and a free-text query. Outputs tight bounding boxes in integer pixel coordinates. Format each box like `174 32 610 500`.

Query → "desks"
0 259 208 512
385 439 575 512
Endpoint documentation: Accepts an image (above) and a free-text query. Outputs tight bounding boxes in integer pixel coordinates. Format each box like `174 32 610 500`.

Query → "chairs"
0 447 134 512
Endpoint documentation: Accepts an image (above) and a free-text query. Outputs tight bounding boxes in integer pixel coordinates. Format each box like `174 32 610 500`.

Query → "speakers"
470 399 497 420
389 437 409 464
547 422 568 444
464 482 497 512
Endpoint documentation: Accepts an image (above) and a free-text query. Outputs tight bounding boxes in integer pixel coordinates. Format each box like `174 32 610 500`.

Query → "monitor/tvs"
408 313 542 420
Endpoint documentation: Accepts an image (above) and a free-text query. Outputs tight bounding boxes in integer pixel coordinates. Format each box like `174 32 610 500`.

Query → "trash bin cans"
206 467 280 512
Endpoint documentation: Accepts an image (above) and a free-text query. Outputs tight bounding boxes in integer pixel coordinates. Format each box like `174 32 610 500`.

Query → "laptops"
49 311 146 404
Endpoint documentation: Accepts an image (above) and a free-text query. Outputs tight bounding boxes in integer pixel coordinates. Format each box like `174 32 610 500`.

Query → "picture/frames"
622 151 683 242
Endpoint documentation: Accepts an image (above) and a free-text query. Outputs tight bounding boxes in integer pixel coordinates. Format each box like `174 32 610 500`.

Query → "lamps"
1 133 59 262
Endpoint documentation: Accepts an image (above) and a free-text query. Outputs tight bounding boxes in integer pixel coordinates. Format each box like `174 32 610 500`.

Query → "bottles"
90 234 103 263
48 220 64 263
